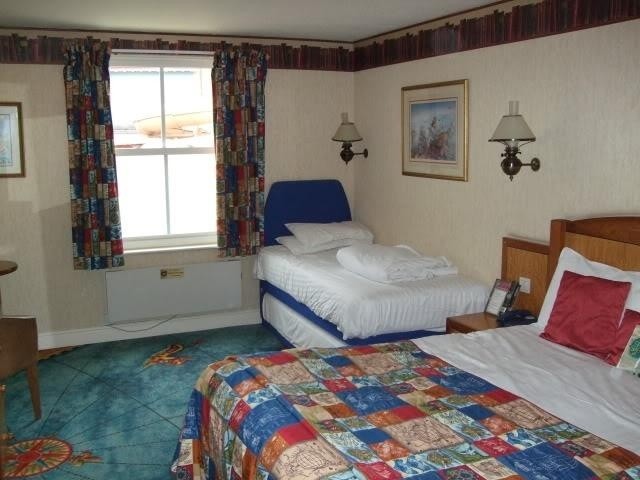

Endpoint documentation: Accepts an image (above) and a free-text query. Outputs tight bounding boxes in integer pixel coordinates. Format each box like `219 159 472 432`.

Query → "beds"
257 178 492 349
199 216 639 479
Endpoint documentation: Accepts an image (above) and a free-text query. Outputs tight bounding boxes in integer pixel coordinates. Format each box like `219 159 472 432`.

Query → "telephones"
498 309 536 327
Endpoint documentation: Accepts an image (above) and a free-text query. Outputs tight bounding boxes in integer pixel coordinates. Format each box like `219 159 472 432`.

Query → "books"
484 278 519 318
618 323 640 373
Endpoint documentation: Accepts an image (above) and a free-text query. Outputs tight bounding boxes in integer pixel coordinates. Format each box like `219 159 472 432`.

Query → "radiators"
105 260 243 324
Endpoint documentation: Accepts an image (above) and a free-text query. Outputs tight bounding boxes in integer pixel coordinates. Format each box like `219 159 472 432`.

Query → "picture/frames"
400 79 468 182
0 101 26 179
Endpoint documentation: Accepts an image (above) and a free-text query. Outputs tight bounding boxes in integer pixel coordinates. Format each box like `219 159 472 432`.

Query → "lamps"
331 112 368 167
488 100 541 182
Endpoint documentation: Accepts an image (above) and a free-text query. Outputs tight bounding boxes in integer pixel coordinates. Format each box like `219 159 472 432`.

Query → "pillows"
274 220 373 255
535 248 640 376
